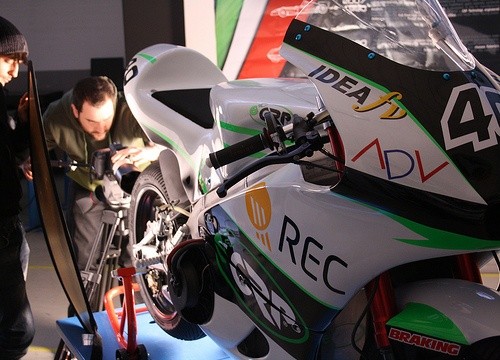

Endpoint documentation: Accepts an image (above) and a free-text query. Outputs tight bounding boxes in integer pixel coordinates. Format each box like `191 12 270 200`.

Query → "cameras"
92 143 141 195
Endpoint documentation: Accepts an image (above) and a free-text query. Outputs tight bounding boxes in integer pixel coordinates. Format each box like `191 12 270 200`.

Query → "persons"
0 16 35 286
23 72 171 318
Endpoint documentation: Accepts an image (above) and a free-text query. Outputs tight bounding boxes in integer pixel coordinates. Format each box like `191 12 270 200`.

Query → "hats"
0 17 28 57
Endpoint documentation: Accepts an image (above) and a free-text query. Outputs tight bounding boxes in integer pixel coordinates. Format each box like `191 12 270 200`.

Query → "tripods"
49 195 145 360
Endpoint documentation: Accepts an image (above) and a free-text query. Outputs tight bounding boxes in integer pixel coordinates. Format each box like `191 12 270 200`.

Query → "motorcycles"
118 0 498 360
